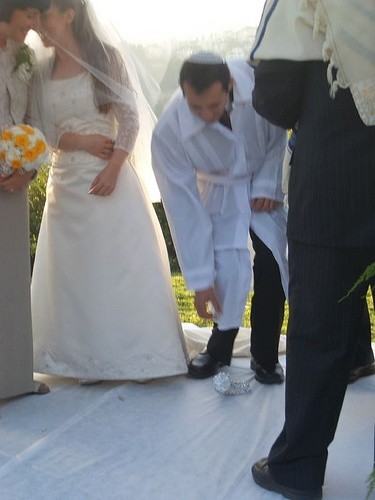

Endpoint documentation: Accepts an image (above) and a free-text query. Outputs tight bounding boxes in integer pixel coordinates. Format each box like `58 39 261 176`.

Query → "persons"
22 0 191 385
246 0 375 500
346 261 375 384
0 0 51 401
151 50 288 386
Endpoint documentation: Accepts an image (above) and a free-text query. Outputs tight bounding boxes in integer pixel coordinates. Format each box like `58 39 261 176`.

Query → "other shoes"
77 378 102 386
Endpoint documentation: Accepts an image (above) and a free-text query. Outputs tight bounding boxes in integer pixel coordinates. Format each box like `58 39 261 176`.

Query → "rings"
11 188 14 192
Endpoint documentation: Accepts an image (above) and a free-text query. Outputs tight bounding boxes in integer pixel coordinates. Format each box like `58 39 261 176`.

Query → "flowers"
12 45 35 83
0 124 49 191
213 370 256 394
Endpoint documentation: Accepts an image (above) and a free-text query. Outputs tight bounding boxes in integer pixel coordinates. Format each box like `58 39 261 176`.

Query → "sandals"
35 381 50 394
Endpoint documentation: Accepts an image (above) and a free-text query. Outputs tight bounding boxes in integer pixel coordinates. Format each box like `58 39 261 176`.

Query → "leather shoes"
187 346 223 378
346 356 375 385
250 356 285 384
251 456 324 500
